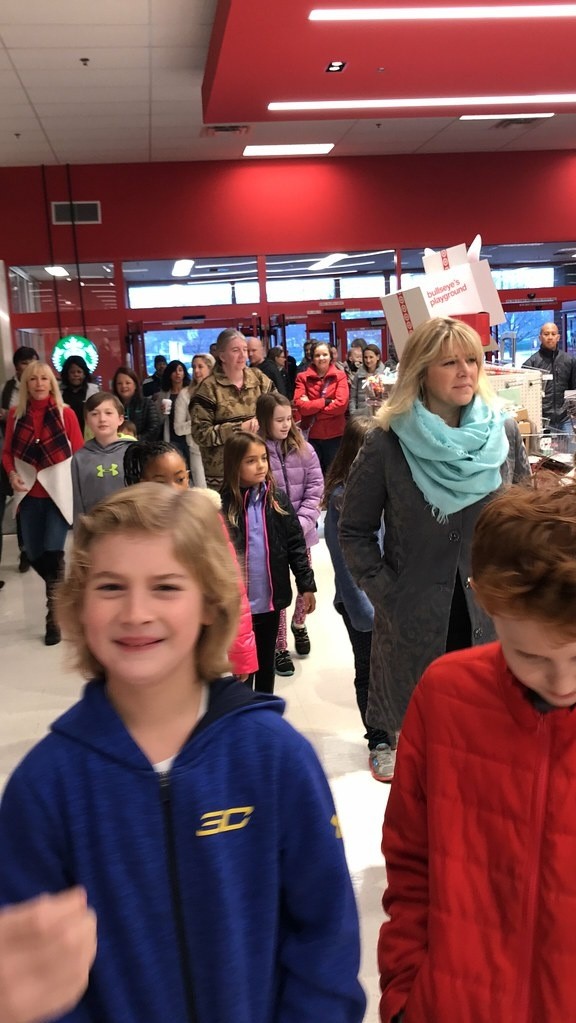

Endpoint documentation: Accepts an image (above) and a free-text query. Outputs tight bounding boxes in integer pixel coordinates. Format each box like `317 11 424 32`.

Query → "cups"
162 398 173 414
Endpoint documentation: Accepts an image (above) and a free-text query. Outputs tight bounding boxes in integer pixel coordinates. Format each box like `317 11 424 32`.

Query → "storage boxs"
381 362 542 438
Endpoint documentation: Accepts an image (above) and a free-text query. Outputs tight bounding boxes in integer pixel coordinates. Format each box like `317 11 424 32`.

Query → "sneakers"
291 621 311 656
368 743 395 781
275 650 295 676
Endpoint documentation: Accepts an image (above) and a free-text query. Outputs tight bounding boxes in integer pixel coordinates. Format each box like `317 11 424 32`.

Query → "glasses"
279 356 285 358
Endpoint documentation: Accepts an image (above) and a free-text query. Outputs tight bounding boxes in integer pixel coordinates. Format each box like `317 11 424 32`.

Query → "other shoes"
45 622 61 645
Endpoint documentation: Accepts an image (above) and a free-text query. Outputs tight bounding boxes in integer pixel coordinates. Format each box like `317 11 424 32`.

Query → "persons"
211 432 318 693
2 359 86 646
0 316 576 490
71 390 140 527
122 439 260 684
336 318 537 732
375 471 576 1022
0 479 370 1023
319 415 396 784
254 392 324 678
